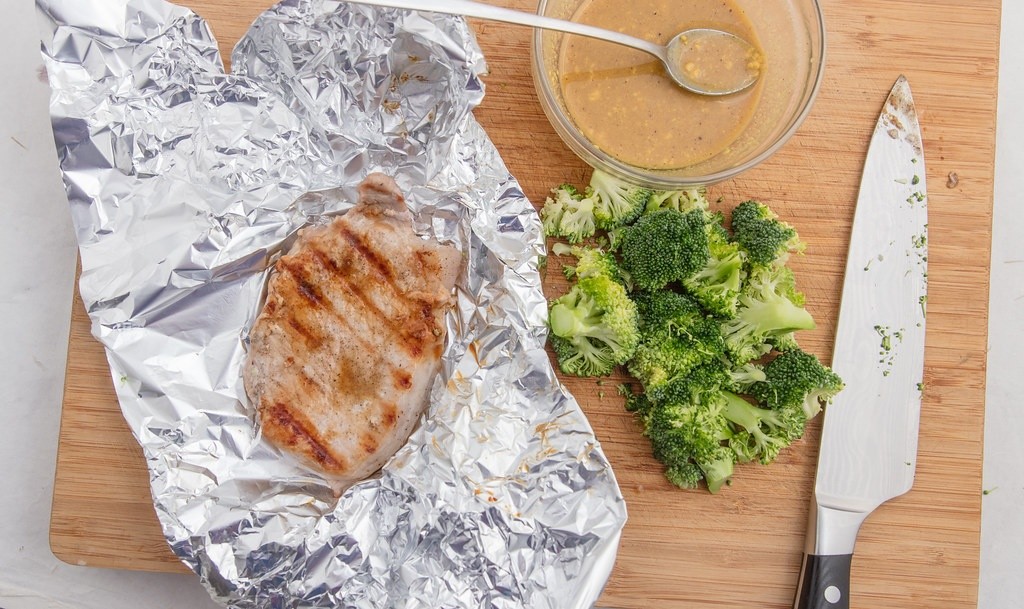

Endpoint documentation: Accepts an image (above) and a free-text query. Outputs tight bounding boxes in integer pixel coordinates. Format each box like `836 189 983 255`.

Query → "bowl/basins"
530 0 826 189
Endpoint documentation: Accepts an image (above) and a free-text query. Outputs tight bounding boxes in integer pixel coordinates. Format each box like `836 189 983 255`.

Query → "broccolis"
536 170 845 494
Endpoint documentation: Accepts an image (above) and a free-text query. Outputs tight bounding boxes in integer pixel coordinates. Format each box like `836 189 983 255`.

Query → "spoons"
333 0 760 96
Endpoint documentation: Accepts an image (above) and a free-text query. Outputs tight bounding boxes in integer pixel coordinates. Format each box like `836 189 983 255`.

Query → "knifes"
790 75 927 609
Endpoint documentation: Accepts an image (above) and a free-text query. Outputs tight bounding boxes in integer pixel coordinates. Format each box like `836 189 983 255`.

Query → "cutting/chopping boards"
49 0 1001 609
